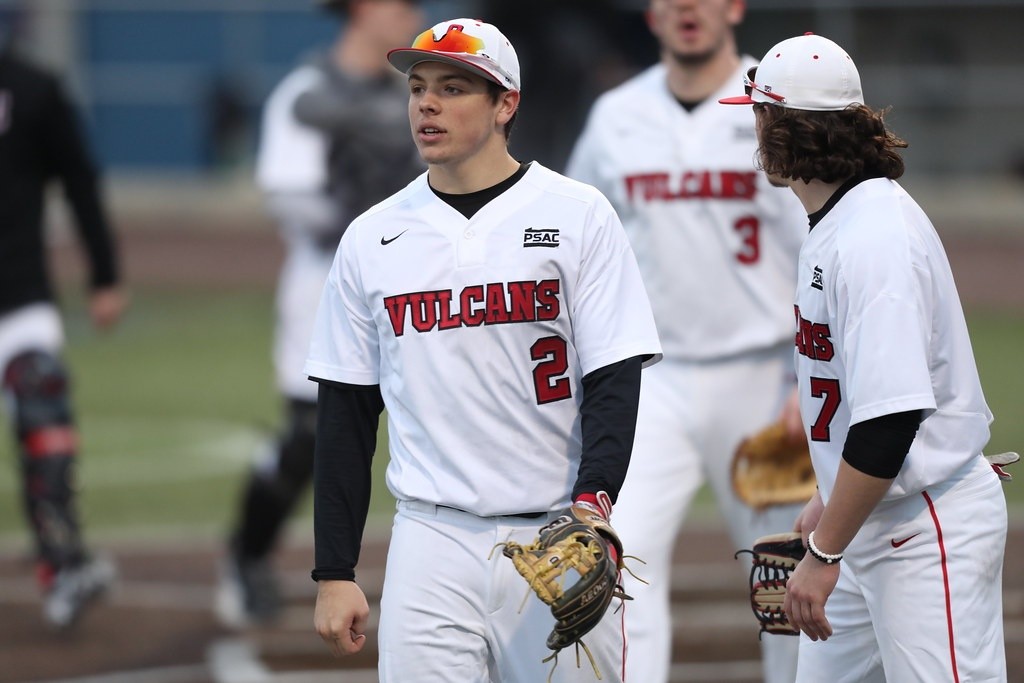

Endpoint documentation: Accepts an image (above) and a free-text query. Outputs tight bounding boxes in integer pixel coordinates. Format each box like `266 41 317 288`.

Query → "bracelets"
804 531 848 565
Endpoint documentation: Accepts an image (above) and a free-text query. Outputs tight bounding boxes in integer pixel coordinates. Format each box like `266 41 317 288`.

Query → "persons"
746 32 1008 683
1 47 128 627
562 0 808 683
209 1 664 624
307 18 664 683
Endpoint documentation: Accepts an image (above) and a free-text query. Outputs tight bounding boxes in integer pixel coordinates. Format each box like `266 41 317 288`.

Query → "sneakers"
209 539 292 637
19 542 118 634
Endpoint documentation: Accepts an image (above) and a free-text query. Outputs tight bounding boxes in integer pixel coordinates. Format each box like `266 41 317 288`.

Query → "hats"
717 31 866 112
386 18 521 95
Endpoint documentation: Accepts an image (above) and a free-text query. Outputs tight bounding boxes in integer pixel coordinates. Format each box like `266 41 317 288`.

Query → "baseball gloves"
498 503 625 652
747 531 807 637
729 418 818 510
